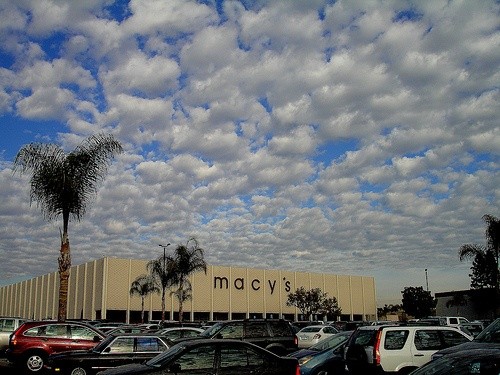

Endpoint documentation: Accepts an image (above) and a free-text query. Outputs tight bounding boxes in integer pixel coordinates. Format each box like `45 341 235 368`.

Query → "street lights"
425 269 429 290
159 243 170 273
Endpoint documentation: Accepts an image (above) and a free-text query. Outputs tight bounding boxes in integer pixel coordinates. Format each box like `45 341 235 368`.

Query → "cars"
286 331 354 375
291 316 490 342
89 320 218 338
44 333 174 375
0 317 32 357
9 320 110 373
296 325 339 348
97 339 301 375
409 318 500 375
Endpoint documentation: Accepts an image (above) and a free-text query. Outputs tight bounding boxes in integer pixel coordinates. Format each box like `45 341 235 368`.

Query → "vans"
373 326 473 375
172 318 298 357
344 325 381 375
